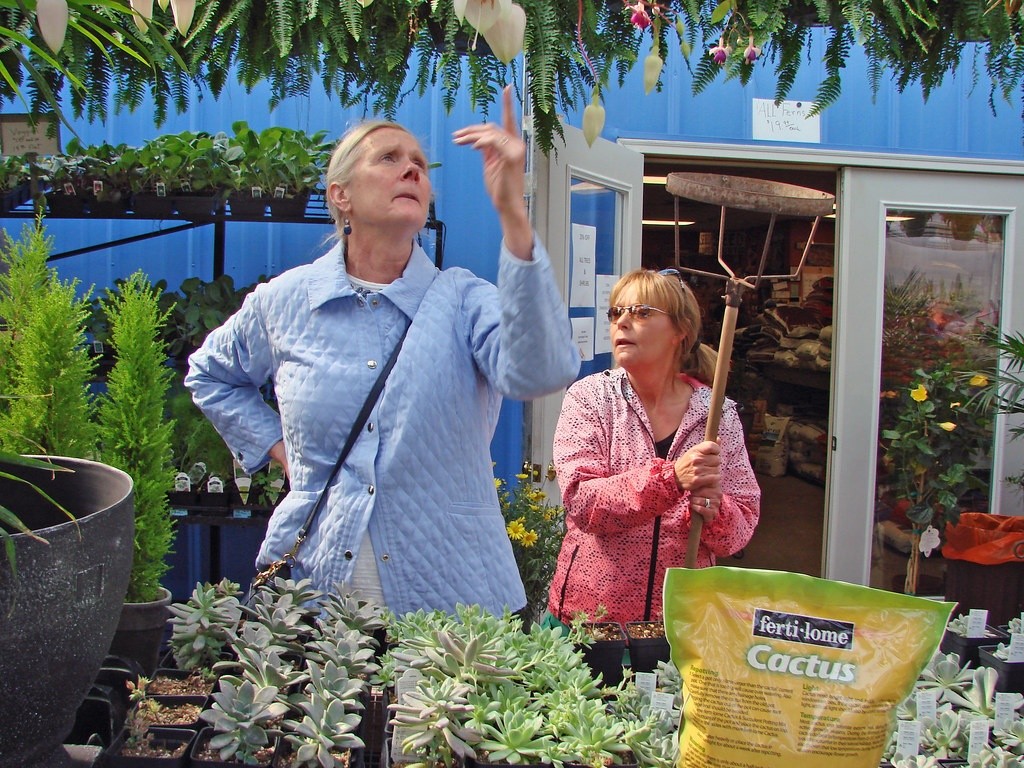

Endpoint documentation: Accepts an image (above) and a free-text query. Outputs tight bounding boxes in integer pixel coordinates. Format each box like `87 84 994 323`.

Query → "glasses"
606 304 670 324
646 268 687 298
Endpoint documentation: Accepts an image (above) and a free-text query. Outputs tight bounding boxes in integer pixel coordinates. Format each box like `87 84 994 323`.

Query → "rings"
500 137 508 145
705 499 710 508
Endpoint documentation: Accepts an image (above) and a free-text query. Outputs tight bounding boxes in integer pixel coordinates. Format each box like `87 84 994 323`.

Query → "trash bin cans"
945 511 1024 629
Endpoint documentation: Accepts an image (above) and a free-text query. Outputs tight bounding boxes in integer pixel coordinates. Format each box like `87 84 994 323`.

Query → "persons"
184 85 582 625
552 270 761 650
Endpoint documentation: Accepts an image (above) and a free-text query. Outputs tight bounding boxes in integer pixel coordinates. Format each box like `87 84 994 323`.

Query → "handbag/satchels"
240 576 325 637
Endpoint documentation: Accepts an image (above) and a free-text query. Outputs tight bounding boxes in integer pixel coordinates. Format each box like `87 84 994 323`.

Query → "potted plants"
0 119 337 218
0 210 669 768
